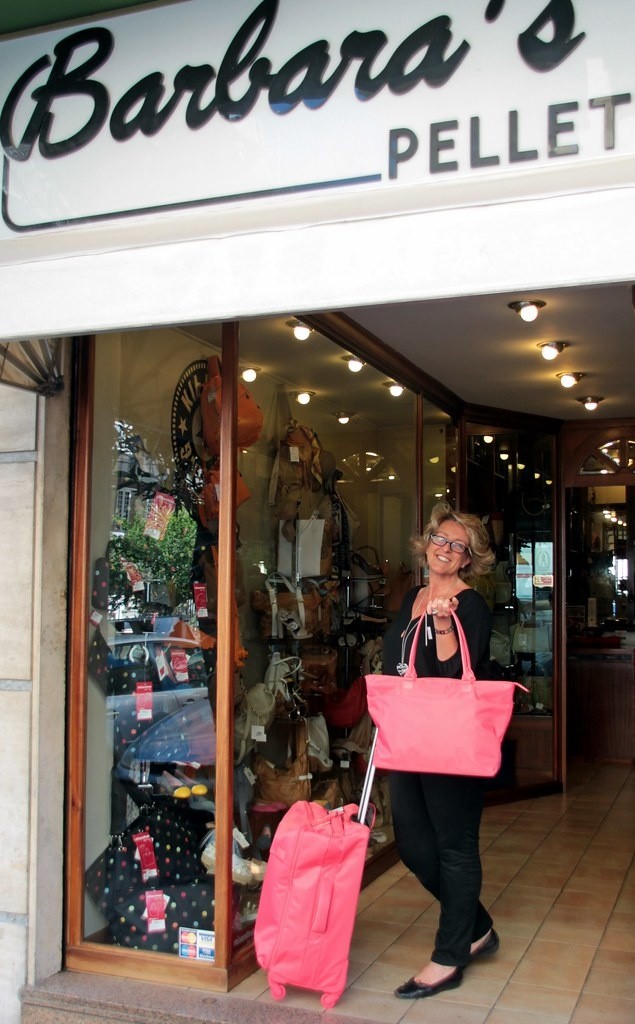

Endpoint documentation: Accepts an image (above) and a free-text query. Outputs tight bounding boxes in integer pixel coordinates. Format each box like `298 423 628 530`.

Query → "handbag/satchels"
201 355 264 456
82 530 262 954
202 450 251 519
365 607 530 778
252 382 394 825
475 498 555 716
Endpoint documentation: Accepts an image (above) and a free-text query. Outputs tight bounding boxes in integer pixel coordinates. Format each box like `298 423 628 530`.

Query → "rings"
432 611 437 614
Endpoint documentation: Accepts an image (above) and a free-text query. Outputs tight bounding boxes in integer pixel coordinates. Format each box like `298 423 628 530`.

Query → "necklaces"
397 586 430 674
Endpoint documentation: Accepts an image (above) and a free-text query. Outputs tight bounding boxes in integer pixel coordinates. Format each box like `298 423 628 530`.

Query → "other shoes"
395 965 462 998
460 928 499 967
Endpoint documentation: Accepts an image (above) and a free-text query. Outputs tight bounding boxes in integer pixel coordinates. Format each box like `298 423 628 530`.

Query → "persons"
379 503 500 1000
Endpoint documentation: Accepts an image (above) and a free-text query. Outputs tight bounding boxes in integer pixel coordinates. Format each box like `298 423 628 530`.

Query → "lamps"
240 366 262 382
556 372 587 388
289 390 316 405
332 410 357 424
382 382 405 397
577 396 604 410
508 300 547 322
341 355 367 373
536 340 571 360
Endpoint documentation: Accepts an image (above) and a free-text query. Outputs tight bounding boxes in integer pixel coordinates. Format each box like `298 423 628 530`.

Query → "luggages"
252 727 378 1009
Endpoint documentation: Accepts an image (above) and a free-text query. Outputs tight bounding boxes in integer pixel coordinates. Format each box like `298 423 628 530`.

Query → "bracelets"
435 625 453 634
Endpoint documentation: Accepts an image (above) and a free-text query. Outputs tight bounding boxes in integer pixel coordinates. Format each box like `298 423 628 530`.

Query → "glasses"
431 534 471 557
